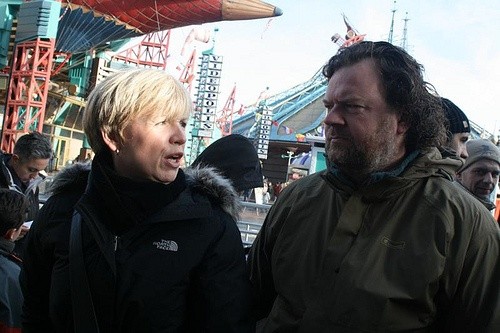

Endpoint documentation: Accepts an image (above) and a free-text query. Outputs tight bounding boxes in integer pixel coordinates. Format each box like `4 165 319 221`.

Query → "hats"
436 96 472 133
456 137 500 174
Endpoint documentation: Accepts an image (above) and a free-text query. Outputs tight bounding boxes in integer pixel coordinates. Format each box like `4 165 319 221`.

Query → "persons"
0 132 51 260
0 188 29 333
435 95 470 168
240 39 500 332
26 68 257 333
190 134 264 193
459 137 500 213
